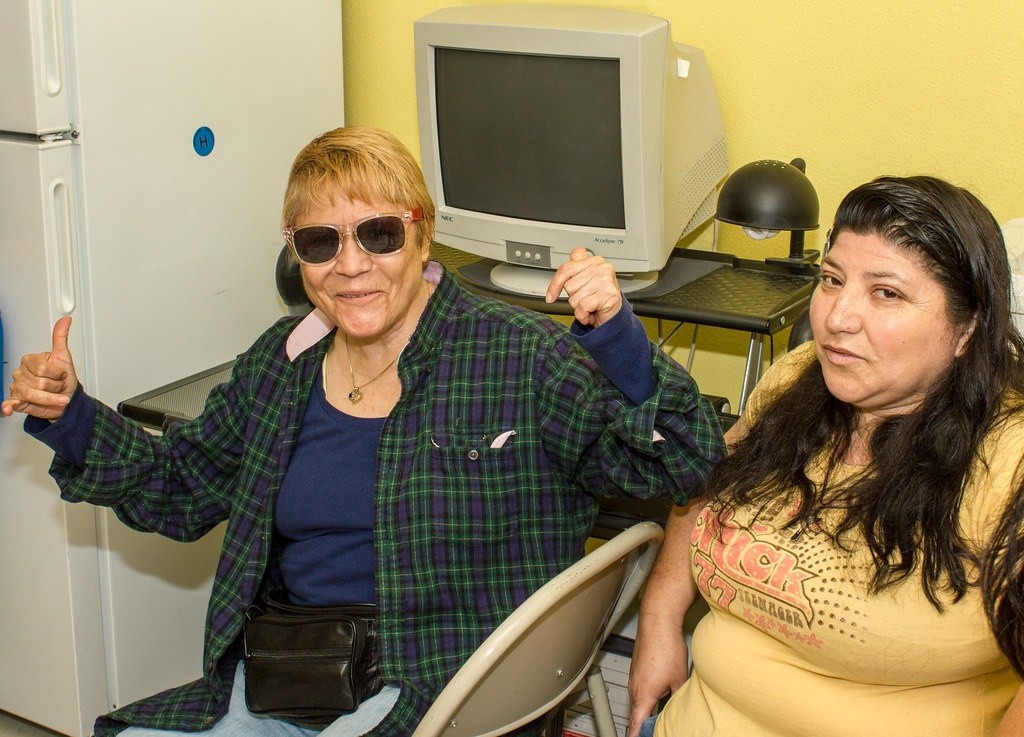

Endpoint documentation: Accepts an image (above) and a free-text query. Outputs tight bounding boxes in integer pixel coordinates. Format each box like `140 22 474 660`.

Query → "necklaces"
344 279 431 405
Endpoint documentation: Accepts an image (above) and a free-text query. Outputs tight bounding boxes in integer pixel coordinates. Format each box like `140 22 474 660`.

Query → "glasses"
284 208 424 266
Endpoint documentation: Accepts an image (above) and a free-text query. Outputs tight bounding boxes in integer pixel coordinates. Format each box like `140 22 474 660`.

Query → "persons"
629 175 1024 737
1 126 730 737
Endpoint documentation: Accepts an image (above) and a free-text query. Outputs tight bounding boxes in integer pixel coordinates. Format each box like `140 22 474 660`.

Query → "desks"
421 243 823 416
116 356 741 542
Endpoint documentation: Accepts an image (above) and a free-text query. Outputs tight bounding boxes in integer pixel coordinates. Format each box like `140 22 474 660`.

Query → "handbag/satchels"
243 586 387 727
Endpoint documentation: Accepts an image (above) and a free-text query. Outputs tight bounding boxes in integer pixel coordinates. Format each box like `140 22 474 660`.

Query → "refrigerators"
0 3 345 737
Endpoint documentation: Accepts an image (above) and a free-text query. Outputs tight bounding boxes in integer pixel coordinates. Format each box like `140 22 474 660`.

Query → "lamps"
713 155 824 268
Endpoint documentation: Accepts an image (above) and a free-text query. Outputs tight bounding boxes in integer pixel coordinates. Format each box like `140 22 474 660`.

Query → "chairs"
413 522 663 737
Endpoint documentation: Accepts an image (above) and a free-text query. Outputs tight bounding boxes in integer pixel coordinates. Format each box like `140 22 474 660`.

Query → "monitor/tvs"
414 0 730 300
